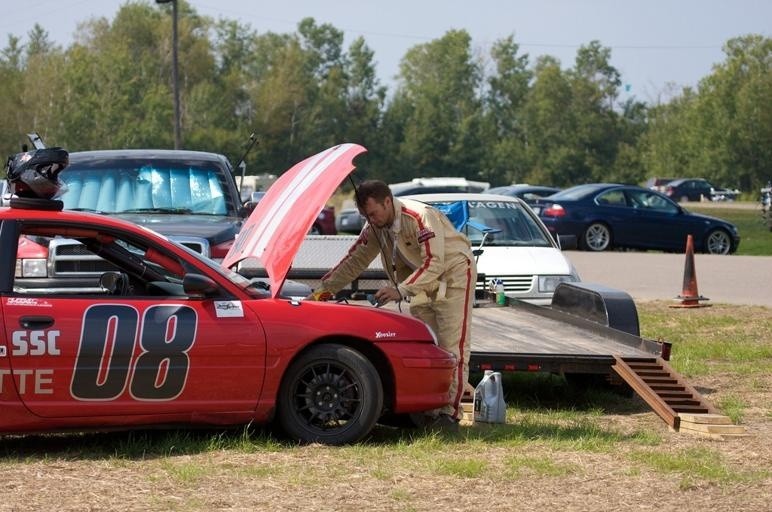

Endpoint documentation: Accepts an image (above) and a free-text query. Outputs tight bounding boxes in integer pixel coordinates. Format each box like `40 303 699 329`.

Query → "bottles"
496 279 505 305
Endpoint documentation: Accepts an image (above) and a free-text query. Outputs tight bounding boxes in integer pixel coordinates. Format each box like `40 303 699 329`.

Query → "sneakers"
427 415 458 433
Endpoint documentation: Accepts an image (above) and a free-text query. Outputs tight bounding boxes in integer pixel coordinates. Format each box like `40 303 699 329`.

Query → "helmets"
5 147 69 199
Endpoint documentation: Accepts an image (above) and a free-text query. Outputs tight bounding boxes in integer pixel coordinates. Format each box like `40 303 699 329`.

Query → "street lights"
154 0 180 150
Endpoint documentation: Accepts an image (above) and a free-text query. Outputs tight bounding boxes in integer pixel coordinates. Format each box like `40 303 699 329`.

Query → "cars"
390 191 581 309
525 183 740 258
0 140 457 448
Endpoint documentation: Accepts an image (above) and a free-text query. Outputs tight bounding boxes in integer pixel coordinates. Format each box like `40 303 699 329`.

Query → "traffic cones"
665 234 714 309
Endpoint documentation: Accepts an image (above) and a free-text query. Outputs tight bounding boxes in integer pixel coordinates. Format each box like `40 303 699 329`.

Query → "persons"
305 179 478 434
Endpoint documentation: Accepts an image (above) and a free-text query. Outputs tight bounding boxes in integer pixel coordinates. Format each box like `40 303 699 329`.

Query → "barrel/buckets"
473 369 506 424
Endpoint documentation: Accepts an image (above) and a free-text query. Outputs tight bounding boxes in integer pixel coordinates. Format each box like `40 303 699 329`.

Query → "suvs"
32 147 252 273
